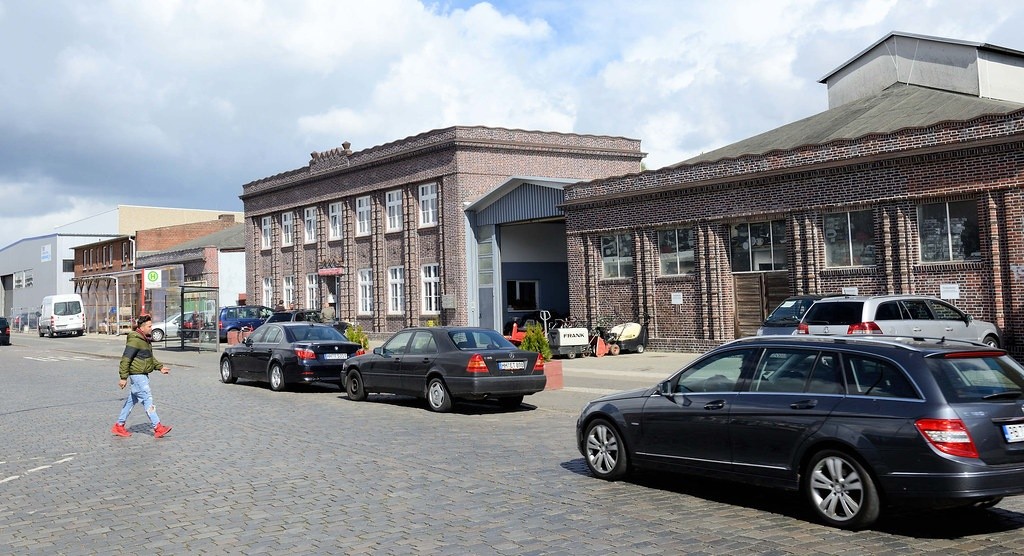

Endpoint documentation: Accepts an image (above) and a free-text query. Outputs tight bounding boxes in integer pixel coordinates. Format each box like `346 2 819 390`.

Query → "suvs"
13 312 39 331
0 316 10 346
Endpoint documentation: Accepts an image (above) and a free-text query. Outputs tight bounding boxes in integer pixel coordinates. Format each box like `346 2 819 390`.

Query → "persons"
275 300 286 312
187 313 208 338
291 303 295 310
112 315 171 439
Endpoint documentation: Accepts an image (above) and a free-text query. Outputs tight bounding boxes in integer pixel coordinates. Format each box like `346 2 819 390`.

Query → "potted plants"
516 321 563 391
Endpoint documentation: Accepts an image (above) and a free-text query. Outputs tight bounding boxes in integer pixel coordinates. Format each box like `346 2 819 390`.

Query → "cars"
264 309 352 334
576 335 1024 530
219 322 366 391
341 327 547 412
151 311 216 343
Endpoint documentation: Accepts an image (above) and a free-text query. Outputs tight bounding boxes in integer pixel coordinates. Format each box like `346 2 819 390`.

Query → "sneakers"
111 422 132 436
153 422 172 438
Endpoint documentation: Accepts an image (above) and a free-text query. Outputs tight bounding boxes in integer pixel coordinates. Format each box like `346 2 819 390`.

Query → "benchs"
607 322 642 341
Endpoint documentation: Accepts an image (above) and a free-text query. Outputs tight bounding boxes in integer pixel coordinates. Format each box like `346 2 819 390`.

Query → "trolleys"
540 310 651 359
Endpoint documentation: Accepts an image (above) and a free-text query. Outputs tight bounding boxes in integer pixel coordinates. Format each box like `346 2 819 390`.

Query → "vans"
219 305 275 339
757 294 1003 349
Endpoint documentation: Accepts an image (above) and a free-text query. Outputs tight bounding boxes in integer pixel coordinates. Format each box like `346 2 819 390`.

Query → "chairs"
249 311 255 317
753 369 804 393
457 342 477 350
304 328 322 340
423 344 438 354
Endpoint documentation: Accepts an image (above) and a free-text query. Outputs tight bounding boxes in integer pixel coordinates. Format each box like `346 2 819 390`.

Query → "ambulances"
36 294 87 338
